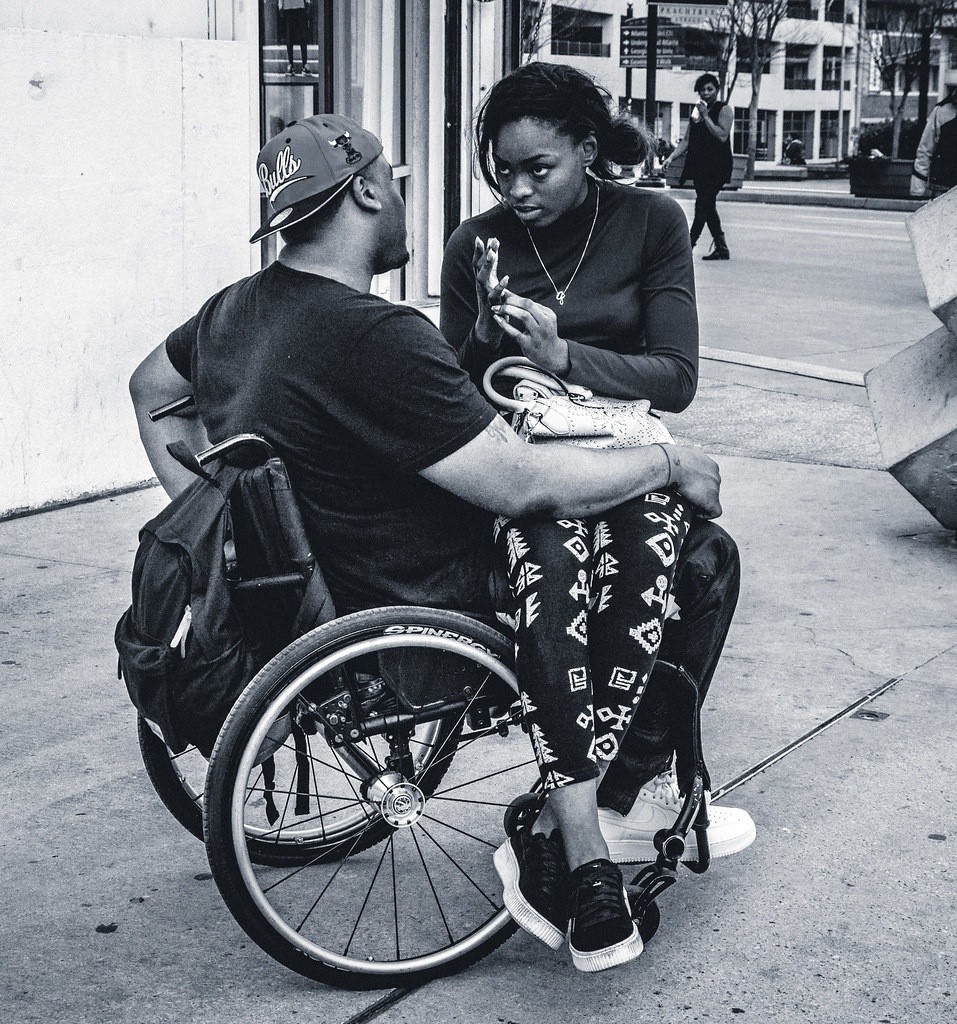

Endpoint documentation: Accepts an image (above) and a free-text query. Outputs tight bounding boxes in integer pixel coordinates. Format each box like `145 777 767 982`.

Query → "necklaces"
521 184 600 305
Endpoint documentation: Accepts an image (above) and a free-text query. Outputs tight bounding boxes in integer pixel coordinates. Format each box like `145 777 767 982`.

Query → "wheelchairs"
136 393 715 993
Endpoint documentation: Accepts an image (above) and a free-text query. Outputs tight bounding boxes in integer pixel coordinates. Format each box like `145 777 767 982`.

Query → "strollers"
782 140 806 166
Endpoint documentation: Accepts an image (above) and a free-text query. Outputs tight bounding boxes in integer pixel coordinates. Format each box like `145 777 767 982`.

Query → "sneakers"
492 828 572 951
568 858 643 972
597 769 756 864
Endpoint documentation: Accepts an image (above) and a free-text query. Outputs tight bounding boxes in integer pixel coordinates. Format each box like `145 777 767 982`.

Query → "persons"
658 138 676 165
441 62 698 972
910 89 957 200
782 134 806 165
129 115 757 864
661 74 733 261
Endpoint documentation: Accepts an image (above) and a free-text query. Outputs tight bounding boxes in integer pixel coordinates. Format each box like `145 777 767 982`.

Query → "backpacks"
115 440 335 770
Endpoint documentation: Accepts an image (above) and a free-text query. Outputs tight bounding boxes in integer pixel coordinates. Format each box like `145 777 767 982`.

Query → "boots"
703 233 729 260
688 232 699 249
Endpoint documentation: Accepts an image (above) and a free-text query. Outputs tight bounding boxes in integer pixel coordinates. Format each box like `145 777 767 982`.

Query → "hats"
248 113 384 245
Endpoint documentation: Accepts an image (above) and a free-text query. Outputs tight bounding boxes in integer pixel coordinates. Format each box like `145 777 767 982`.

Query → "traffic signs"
619 25 687 70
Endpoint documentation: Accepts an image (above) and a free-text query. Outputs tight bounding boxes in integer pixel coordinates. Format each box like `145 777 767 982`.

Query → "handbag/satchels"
482 356 677 450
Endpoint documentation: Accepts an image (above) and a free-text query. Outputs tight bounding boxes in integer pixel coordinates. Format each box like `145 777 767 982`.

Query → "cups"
691 99 707 120
871 148 883 157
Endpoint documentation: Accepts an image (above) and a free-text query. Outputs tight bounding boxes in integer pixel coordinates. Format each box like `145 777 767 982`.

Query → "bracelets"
651 443 671 490
